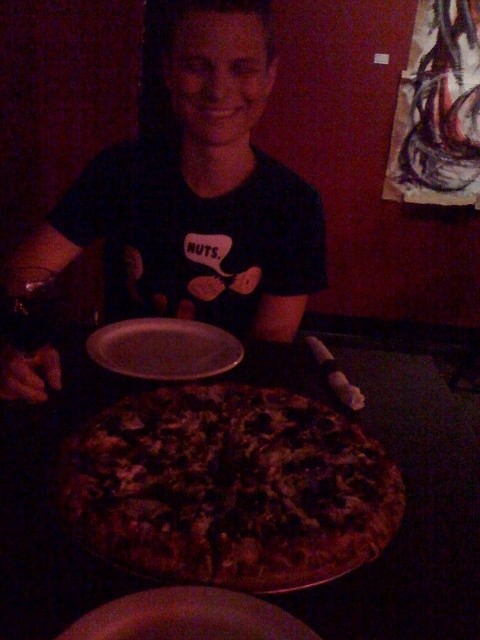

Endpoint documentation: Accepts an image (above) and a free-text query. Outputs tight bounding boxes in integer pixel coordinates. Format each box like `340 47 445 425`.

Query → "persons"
1 0 333 403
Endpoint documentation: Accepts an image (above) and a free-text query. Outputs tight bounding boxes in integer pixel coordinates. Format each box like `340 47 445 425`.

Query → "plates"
52 581 321 639
84 317 247 383
40 475 350 597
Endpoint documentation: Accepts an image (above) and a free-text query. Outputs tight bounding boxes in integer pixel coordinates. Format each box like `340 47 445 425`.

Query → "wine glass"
0 262 62 368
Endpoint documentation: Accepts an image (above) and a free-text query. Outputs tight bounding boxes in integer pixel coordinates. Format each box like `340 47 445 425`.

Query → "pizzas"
56 384 406 591
